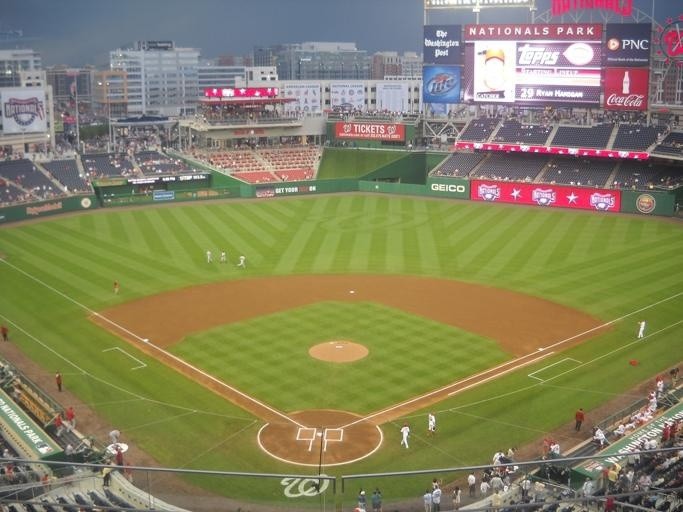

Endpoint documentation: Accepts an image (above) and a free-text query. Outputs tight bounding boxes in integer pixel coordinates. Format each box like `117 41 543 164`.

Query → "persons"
353 368 682 511
203 105 682 192
1 105 202 206
636 319 646 339
207 250 213 264
113 279 121 294
220 250 226 264
1 367 133 493
0 326 9 341
236 254 246 269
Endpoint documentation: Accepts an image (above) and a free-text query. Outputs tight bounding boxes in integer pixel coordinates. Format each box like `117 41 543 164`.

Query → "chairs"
0 362 145 512
1 109 683 208
339 114 341 118
501 387 682 510
